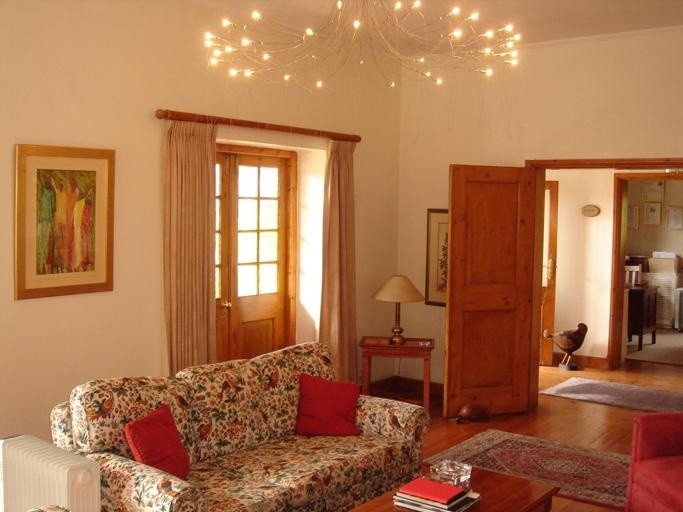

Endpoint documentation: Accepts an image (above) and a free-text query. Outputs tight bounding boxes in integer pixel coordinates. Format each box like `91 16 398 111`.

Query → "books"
394 476 480 512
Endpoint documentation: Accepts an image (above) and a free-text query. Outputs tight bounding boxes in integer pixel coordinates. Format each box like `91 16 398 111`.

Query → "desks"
342 464 558 512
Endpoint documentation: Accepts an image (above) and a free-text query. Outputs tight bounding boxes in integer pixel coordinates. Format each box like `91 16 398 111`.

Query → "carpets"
424 428 631 509
537 376 683 414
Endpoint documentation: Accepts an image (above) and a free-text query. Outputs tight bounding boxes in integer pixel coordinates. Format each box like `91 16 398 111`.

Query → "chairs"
623 263 643 285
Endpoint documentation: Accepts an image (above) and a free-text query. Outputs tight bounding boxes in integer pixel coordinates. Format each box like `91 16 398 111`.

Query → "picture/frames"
666 206 683 230
425 208 448 307
15 143 114 301
641 179 664 202
643 202 662 225
628 206 640 230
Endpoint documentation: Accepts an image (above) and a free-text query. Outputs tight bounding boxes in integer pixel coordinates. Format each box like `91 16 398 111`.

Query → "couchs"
50 342 430 512
625 411 682 512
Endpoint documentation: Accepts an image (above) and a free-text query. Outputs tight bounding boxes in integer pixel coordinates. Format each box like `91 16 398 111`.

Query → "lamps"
372 275 426 344
199 1 521 92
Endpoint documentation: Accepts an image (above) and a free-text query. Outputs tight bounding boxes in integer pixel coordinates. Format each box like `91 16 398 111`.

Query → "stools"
358 335 435 414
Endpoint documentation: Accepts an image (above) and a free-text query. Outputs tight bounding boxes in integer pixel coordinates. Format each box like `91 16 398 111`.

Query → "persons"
37 174 92 273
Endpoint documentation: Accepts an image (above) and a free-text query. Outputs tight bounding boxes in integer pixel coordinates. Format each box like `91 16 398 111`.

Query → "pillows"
296 372 360 437
124 404 190 480
